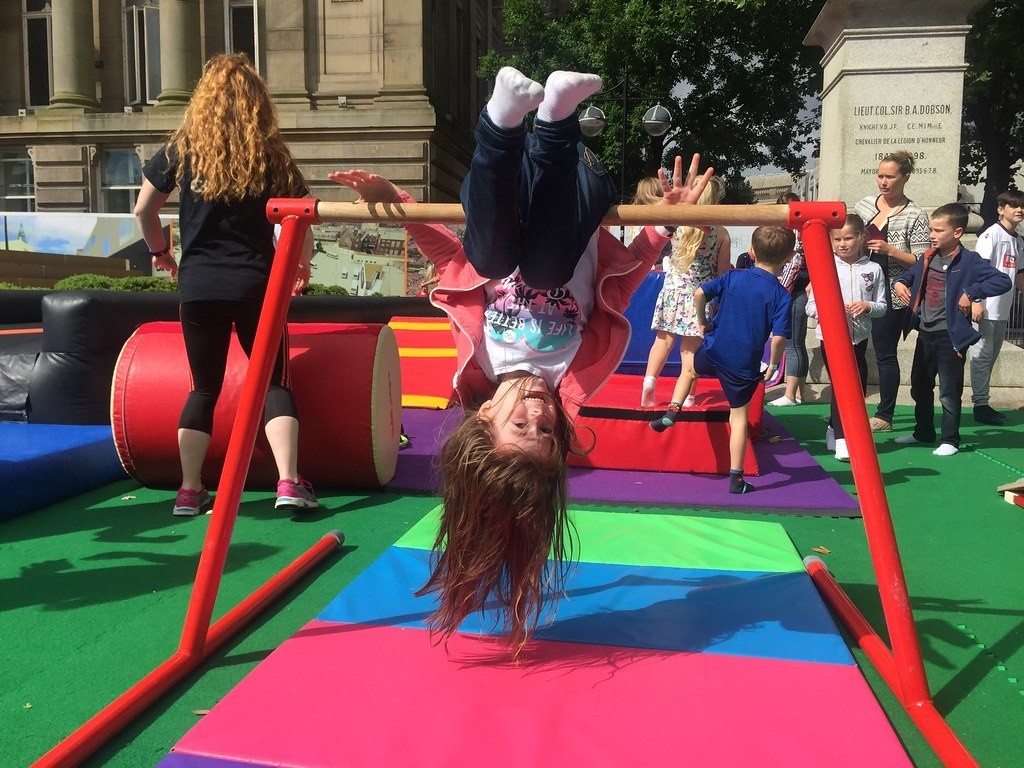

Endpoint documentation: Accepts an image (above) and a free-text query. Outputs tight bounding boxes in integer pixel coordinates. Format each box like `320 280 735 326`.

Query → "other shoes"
869 416 891 431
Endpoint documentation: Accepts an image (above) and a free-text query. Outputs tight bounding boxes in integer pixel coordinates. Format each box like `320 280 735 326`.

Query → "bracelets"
769 364 778 370
664 225 676 234
148 244 170 257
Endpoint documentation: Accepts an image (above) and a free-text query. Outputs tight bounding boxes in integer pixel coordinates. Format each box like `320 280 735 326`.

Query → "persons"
891 202 1012 456
969 188 1024 424
766 193 813 408
323 63 715 666
133 51 322 516
804 151 932 436
640 174 731 404
805 212 888 463
647 224 795 495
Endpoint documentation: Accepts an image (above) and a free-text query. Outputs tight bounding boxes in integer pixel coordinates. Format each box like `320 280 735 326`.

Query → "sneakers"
172 483 211 515
274 473 318 509
